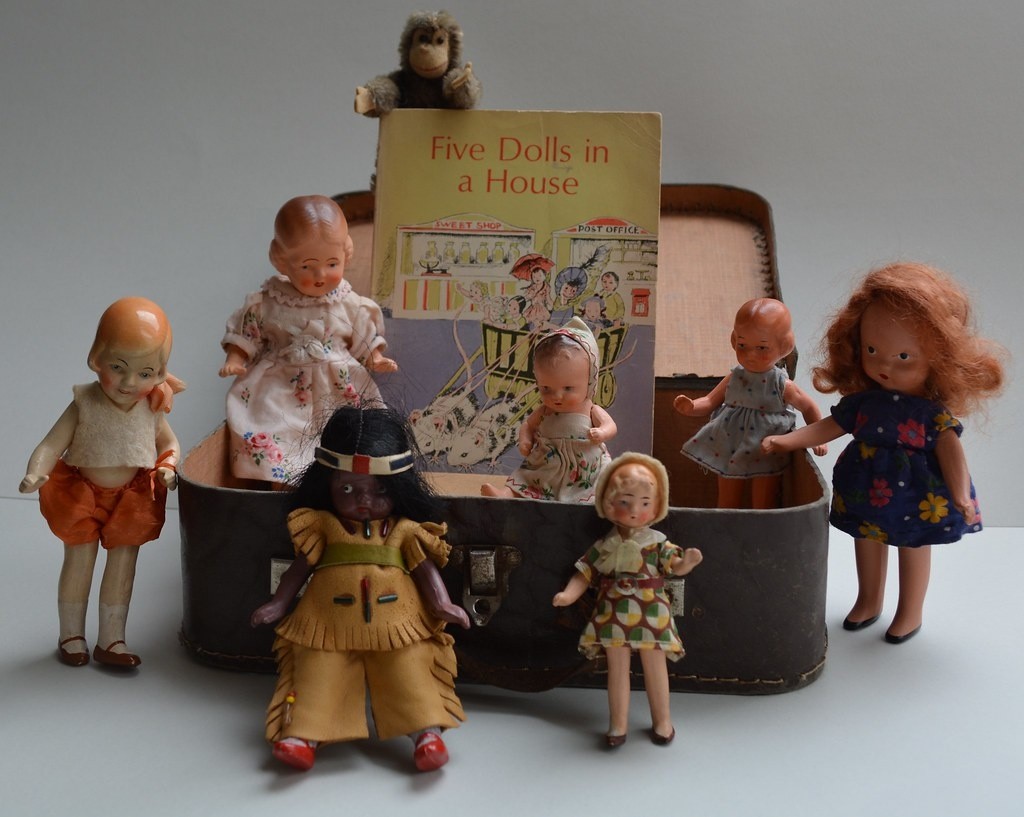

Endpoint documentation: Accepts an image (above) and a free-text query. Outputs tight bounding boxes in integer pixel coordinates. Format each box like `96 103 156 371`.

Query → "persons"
18 295 181 667
479 315 618 505
552 451 704 750
673 297 828 508
218 196 399 491
761 262 1001 644
250 406 472 773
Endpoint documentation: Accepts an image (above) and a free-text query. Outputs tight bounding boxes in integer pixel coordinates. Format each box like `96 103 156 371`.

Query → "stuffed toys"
353 9 481 193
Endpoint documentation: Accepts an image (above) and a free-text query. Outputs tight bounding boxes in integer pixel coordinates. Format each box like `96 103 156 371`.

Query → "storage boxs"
177 184 829 695
